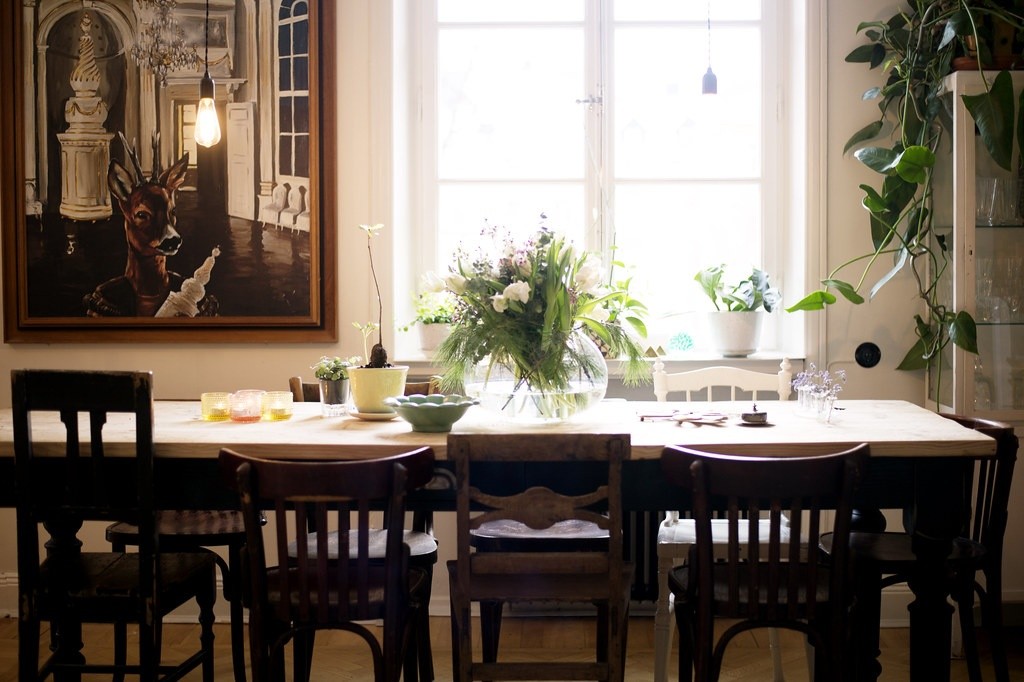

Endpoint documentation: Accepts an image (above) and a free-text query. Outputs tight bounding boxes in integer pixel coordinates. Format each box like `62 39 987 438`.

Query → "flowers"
413 216 648 396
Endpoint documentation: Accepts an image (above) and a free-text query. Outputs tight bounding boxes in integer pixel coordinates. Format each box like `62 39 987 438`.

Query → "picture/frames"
0 0 340 348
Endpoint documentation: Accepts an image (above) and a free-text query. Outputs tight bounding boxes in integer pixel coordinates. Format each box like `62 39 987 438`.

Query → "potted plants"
695 263 782 357
309 356 358 405
782 0 1024 373
345 224 410 420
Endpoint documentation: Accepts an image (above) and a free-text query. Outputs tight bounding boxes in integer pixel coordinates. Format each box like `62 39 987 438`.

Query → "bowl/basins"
384 395 480 432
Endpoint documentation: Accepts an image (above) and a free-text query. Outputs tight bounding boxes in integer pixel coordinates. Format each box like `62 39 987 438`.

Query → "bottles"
973 354 995 410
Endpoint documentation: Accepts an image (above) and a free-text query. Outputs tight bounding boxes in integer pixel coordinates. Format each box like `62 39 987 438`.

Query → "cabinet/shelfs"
916 69 1024 602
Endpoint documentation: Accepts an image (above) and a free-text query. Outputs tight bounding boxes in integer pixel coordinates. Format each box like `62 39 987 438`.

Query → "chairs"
12 367 1024 682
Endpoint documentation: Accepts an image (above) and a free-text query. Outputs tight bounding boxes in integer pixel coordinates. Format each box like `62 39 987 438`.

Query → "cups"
232 389 265 422
974 257 992 317
998 178 1020 224
201 392 232 421
975 176 998 225
262 391 293 421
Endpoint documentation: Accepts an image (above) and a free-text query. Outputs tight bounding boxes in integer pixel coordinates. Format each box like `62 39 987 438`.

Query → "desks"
0 396 996 682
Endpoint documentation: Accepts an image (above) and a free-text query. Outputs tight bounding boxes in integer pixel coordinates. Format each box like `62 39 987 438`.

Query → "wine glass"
995 257 1022 321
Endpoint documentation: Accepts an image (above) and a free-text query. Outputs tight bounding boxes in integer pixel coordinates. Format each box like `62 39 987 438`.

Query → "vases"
516 329 608 423
